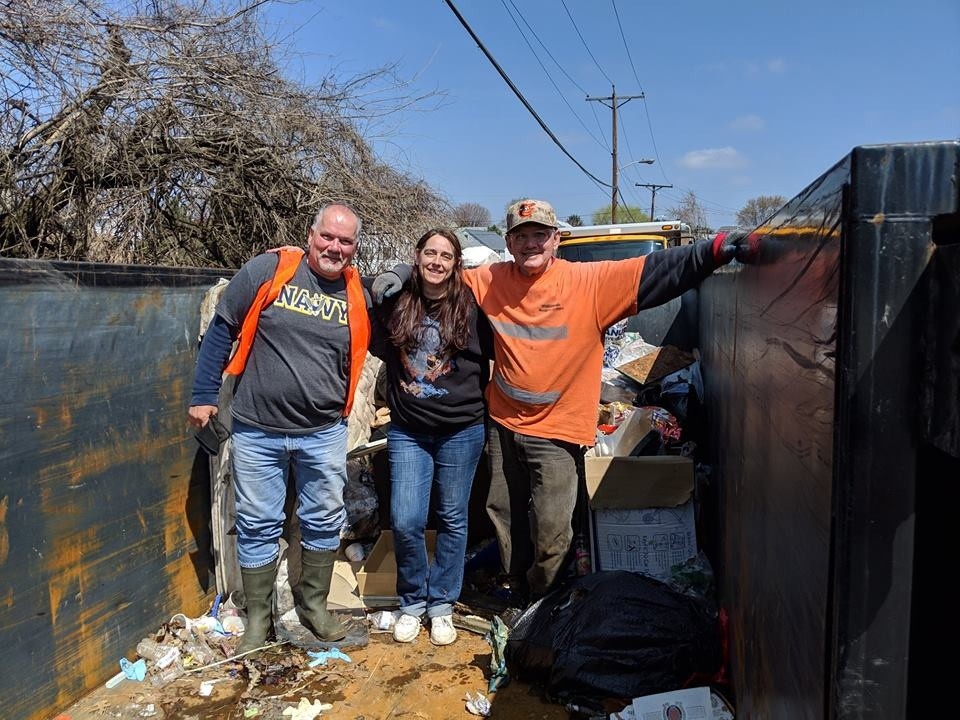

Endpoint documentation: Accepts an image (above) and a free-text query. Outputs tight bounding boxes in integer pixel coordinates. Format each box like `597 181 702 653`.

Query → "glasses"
509 228 555 240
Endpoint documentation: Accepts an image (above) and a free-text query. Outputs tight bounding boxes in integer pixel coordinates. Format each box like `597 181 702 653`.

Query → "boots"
298 545 347 642
235 557 278 662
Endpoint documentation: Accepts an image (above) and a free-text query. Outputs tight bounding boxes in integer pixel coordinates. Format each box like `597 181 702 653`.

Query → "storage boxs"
585 445 698 585
356 527 449 596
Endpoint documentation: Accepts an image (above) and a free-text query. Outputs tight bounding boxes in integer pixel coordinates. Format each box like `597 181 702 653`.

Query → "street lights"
611 159 655 223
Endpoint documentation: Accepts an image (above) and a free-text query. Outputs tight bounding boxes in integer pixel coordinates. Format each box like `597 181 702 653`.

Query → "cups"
170 614 195 635
149 663 184 688
345 543 366 563
223 590 247 610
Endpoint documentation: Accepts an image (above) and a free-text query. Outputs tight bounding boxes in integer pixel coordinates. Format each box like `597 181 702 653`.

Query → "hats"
505 199 559 235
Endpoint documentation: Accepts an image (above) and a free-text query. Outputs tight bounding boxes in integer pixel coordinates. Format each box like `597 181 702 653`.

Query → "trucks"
556 222 696 263
454 225 516 270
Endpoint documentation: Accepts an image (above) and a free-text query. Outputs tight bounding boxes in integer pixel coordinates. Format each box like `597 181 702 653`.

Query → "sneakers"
429 614 457 646
392 613 424 643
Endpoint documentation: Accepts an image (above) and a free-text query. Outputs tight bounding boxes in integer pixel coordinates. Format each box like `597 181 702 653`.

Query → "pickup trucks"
701 225 741 241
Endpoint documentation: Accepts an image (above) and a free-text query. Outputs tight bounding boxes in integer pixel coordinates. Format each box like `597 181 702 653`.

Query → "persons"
186 203 372 662
365 229 496 646
368 197 748 609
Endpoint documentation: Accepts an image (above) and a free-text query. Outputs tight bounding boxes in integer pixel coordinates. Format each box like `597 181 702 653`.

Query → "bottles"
366 611 403 630
575 521 590 575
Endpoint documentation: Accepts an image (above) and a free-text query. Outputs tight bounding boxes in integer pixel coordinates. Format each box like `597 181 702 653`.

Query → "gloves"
371 271 403 306
714 229 766 263
193 415 230 457
